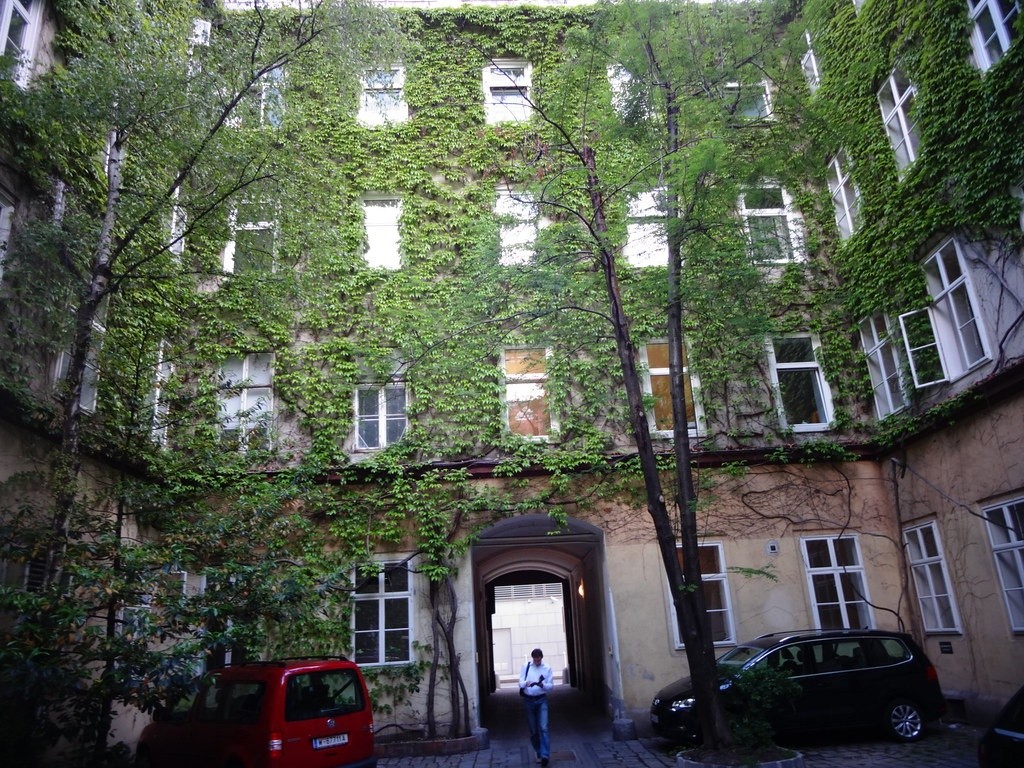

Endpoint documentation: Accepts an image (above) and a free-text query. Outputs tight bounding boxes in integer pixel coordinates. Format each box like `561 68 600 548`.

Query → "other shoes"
536 752 549 768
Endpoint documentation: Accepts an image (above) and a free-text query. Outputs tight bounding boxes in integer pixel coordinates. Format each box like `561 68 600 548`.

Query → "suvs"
649 626 945 744
135 655 378 768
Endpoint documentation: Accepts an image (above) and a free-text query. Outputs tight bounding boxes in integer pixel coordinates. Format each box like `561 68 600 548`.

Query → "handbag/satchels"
520 688 524 696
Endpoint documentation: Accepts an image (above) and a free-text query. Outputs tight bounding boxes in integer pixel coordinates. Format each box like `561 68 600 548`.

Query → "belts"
526 693 545 701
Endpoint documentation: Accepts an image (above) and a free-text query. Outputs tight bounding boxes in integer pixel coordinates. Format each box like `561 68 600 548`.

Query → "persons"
519 649 553 767
